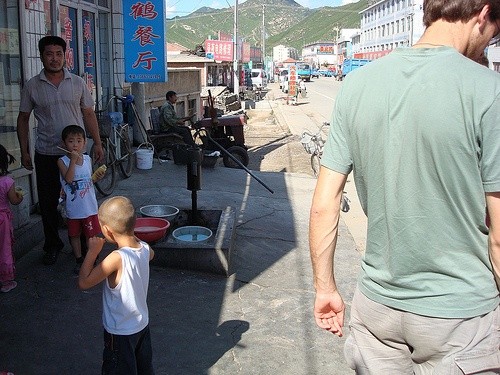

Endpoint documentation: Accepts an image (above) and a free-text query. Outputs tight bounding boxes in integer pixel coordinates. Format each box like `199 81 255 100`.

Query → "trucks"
277 58 372 93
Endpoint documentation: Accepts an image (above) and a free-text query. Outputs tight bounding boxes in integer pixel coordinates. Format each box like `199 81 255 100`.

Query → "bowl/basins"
139 205 180 221
134 217 170 242
171 225 212 244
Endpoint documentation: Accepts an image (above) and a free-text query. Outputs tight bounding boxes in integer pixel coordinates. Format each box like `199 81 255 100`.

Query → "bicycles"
85 95 136 196
301 122 350 212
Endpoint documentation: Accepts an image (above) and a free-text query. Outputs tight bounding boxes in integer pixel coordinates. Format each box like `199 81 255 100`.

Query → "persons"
57 125 101 263
79 195 155 375
0 144 22 293
159 90 197 148
17 36 104 265
310 0 500 375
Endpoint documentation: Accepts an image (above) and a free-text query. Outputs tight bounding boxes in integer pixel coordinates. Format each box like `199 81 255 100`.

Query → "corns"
92 164 108 180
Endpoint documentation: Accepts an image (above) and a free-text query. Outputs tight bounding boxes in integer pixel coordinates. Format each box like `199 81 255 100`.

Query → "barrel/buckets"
135 142 154 169
171 144 192 165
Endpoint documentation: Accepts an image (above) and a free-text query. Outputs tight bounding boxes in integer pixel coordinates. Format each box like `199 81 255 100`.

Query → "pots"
203 150 219 167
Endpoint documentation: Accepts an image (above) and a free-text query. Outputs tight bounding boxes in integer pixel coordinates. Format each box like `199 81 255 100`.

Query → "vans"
250 69 267 89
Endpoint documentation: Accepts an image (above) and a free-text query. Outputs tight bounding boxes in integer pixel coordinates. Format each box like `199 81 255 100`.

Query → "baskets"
83 114 112 139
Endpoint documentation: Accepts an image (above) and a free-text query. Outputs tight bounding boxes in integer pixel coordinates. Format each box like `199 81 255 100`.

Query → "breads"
16 191 24 196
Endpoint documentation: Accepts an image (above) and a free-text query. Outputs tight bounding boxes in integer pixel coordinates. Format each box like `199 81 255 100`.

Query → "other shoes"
42 250 59 266
0 280 18 293
75 261 84 274
